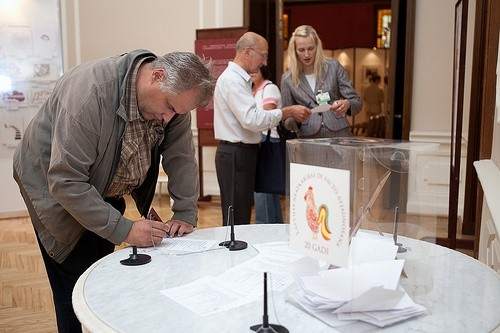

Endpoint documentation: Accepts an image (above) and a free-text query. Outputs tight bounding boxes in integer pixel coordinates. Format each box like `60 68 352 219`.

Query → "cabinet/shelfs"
473 46 500 275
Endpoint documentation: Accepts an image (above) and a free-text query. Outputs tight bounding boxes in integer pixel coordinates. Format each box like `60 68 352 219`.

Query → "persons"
214 31 312 227
280 25 363 139
13 47 216 333
361 75 384 117
250 64 283 224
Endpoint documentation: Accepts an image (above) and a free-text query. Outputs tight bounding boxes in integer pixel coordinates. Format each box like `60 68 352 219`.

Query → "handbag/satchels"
255 126 286 193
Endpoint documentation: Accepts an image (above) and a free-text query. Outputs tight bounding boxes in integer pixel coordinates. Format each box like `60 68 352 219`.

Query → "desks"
71 224 500 333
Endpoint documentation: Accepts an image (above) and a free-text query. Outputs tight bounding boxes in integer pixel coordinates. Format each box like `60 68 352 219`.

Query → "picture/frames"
344 65 351 80
362 65 381 84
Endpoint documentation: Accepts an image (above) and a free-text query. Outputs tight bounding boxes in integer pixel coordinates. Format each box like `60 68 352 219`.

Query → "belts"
220 140 259 148
104 196 122 200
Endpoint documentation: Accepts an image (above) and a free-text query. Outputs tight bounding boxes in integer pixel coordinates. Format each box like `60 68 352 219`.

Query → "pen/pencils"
307 102 311 107
150 213 171 237
230 206 234 242
262 271 269 328
393 205 400 241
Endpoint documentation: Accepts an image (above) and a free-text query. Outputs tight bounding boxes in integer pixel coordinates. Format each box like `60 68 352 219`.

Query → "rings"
339 106 342 112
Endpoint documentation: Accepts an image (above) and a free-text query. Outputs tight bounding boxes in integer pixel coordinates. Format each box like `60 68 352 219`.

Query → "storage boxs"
284 136 442 333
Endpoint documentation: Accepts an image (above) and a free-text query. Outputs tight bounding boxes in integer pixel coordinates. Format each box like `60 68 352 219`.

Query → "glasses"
250 48 266 59
